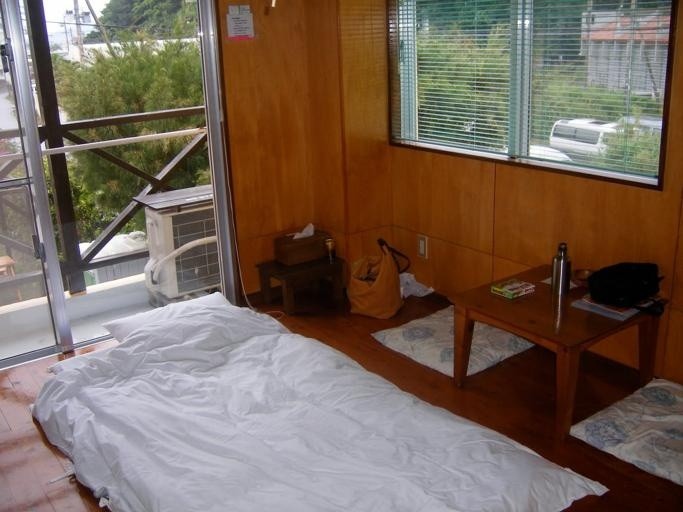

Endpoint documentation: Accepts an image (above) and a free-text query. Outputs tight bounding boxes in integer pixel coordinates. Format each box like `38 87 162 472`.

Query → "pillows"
101 291 230 344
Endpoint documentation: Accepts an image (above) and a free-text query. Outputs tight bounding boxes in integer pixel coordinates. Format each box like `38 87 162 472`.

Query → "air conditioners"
144 205 221 302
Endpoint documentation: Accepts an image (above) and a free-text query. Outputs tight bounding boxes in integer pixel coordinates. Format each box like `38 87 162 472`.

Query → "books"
571 293 662 321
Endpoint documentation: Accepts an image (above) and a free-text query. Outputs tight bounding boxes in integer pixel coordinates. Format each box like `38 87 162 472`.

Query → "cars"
528 144 573 163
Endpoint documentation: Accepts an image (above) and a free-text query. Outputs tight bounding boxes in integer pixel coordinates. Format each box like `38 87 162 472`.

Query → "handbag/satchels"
586 262 664 308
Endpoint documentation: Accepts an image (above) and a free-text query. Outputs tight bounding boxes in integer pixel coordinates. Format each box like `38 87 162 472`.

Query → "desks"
447 265 660 435
257 257 343 315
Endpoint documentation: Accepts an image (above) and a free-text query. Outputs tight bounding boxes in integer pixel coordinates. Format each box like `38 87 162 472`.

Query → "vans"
617 114 661 137
549 118 638 161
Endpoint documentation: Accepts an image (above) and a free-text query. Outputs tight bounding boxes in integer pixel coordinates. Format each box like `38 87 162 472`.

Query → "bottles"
310 238 345 318
551 242 571 295
552 295 565 337
